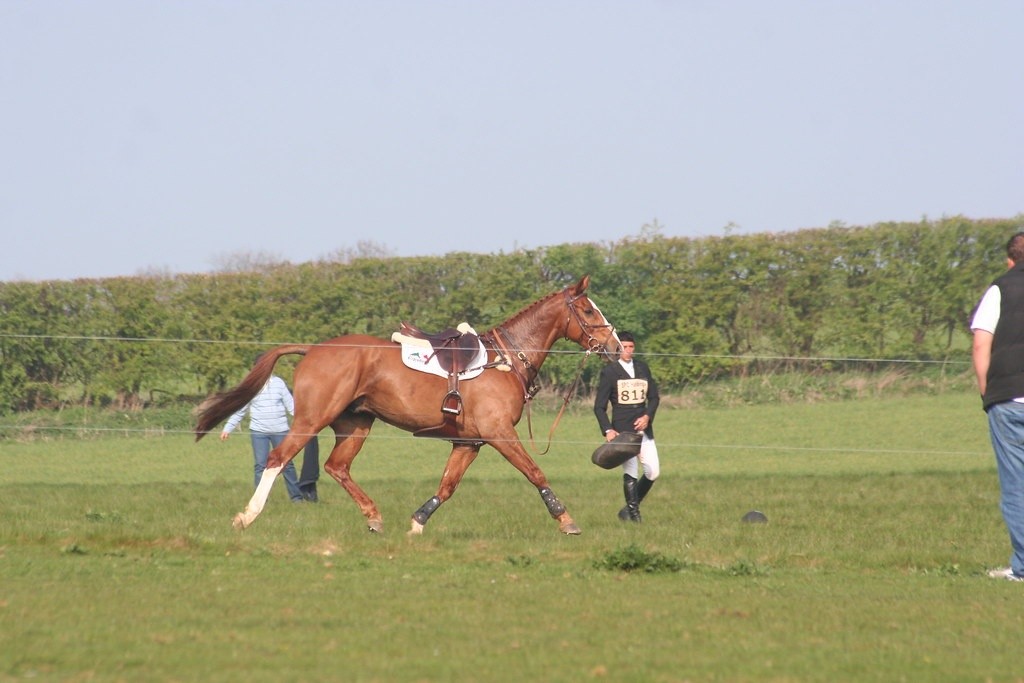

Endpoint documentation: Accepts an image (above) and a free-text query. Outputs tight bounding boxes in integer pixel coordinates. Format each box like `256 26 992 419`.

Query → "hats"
617 331 634 342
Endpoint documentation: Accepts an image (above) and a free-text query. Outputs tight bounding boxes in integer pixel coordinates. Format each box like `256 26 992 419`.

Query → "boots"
616 474 655 521
624 473 641 523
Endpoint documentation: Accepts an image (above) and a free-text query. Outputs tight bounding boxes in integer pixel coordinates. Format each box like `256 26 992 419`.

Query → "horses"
193 272 624 538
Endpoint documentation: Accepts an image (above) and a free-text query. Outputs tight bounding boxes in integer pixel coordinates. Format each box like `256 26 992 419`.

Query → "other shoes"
989 567 1024 583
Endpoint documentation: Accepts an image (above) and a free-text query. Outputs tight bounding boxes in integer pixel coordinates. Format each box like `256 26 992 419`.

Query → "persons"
220 354 302 502
969 232 1024 581
595 331 659 522
